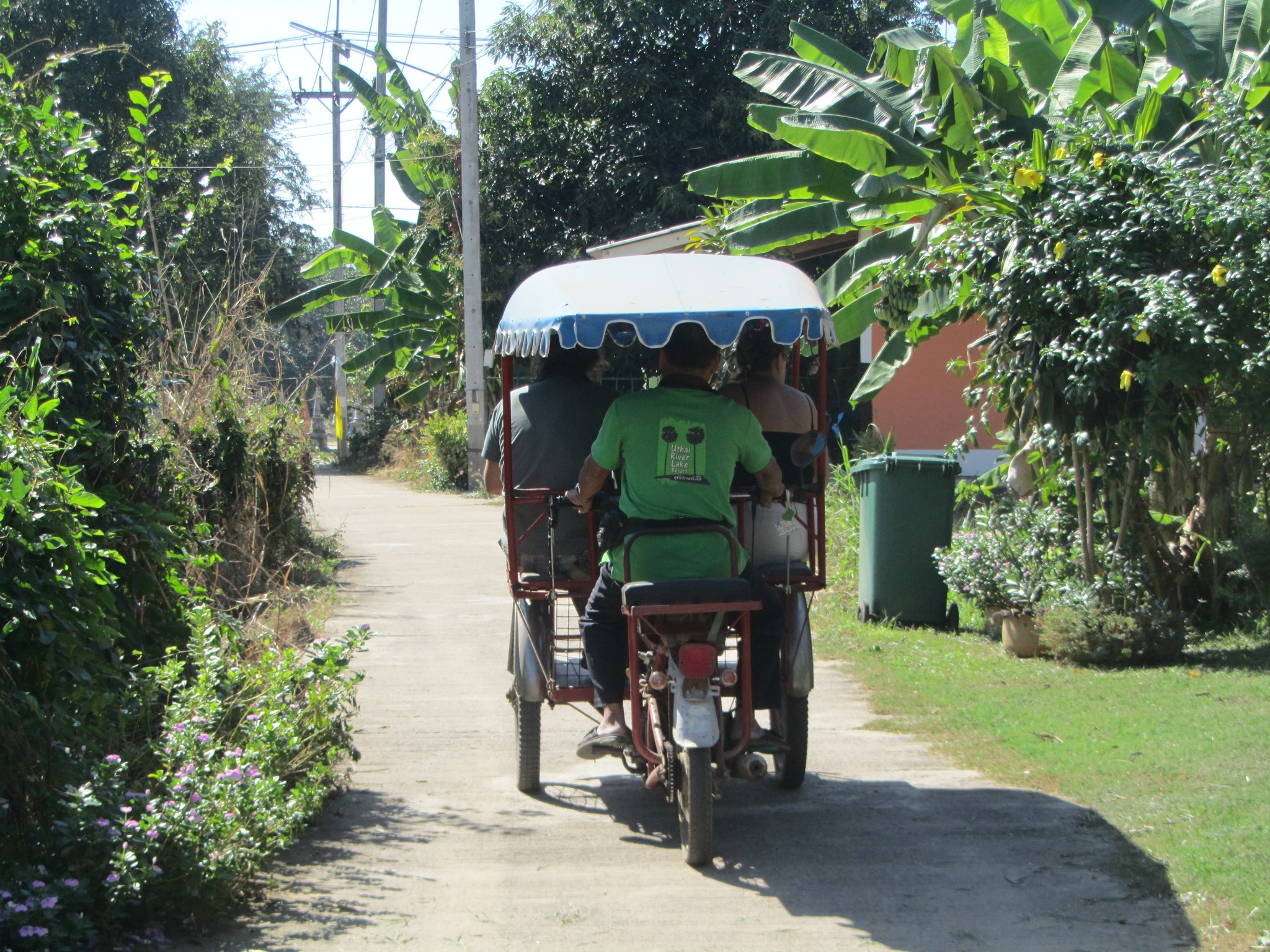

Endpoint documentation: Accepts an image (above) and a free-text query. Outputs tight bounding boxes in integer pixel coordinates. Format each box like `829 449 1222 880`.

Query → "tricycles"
495 250 841 865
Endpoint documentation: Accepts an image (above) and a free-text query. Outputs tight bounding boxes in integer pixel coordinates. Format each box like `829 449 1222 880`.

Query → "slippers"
734 729 789 753
575 725 628 760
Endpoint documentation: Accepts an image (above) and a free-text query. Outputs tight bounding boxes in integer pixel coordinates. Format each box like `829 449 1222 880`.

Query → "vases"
984 606 1010 640
1003 614 1039 657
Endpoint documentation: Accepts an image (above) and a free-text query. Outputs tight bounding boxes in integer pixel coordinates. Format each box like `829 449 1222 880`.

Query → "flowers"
932 499 1089 616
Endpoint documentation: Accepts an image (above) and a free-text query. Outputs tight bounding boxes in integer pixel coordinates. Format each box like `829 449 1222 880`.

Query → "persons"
562 321 787 760
480 333 623 669
714 321 830 571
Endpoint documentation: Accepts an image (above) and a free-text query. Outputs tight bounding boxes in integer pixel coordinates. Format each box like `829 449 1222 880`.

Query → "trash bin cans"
850 453 964 624
